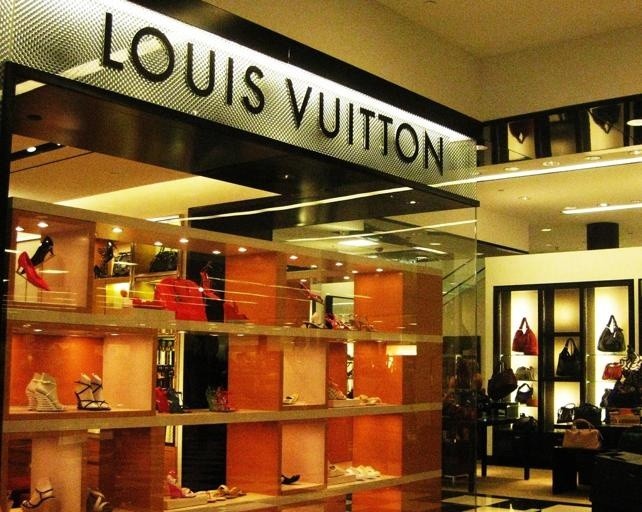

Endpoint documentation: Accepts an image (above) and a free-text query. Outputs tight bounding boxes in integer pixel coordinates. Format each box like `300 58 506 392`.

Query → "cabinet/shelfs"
492 279 636 470
1 196 442 512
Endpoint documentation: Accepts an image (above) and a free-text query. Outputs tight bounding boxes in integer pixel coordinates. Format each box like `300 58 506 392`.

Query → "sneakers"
328 462 380 480
283 393 299 404
281 473 300 484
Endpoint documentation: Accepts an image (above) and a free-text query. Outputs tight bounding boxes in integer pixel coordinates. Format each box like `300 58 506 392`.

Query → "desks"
443 418 532 493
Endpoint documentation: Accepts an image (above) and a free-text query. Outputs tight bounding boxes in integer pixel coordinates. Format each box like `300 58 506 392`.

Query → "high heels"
167 475 194 497
20 488 56 512
103 240 117 262
94 264 107 278
25 372 65 411
223 301 254 324
295 281 375 331
200 272 220 299
20 235 53 274
17 251 50 291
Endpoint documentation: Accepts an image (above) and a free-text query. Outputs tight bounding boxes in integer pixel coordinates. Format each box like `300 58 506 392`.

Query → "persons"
468 361 482 389
449 360 476 389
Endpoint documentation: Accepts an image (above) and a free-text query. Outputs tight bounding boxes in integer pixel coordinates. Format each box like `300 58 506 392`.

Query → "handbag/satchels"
557 338 582 376
515 384 533 404
512 318 539 355
113 253 130 275
442 359 491 426
601 352 642 424
154 278 208 321
488 360 517 401
562 419 602 451
513 414 538 435
444 437 470 466
514 366 536 381
491 403 518 423
556 403 601 426
150 251 176 272
598 316 626 352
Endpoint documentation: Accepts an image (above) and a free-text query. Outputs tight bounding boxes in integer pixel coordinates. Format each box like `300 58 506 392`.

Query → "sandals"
154 385 183 413
327 378 347 400
197 485 245 502
87 488 112 512
353 394 380 406
206 387 237 411
73 374 111 410
